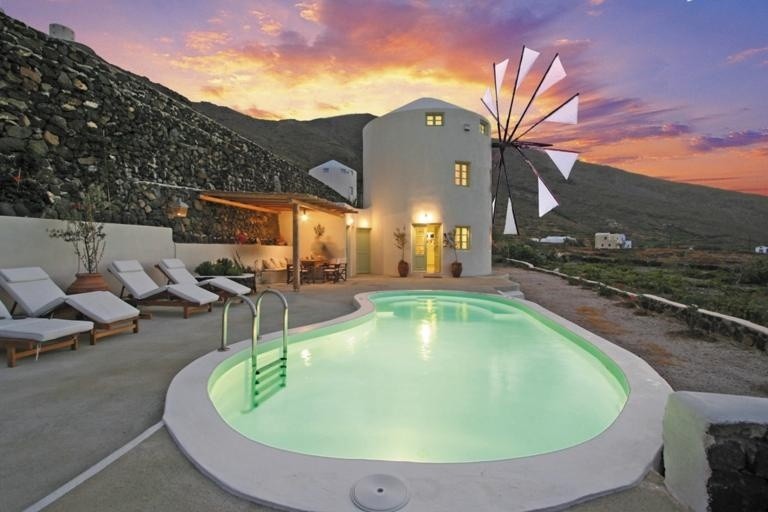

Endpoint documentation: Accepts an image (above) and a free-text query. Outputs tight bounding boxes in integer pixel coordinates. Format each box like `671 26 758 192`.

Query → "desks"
301 260 320 283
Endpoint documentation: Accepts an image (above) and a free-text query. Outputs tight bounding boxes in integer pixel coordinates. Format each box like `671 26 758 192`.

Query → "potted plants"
392 226 408 277
443 230 463 278
46 184 119 318
193 256 256 295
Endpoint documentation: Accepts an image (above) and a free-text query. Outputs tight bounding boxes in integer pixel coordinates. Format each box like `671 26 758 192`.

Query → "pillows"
263 256 289 269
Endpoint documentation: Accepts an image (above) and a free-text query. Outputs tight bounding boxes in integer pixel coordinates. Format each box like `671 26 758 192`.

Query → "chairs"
108 258 254 317
323 263 346 284
1 267 153 367
287 264 313 285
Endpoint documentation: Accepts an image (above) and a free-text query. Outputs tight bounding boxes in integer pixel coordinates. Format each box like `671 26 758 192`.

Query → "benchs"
262 268 347 284
